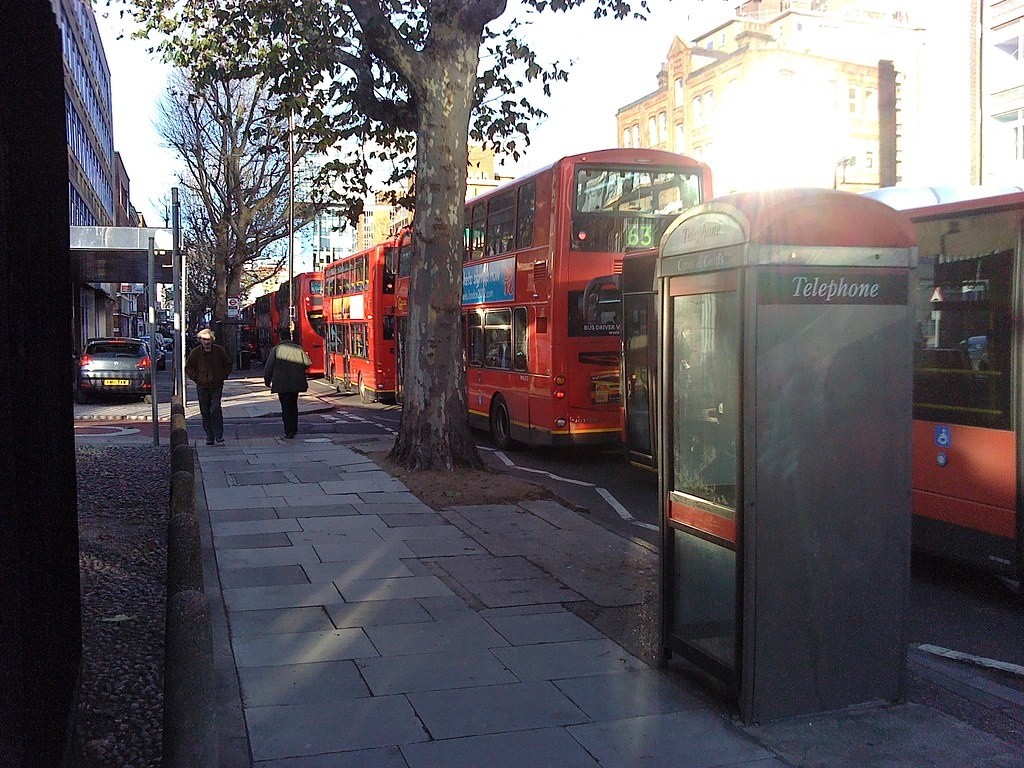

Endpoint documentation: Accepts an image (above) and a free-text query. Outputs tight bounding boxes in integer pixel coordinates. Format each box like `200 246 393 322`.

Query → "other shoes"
216 436 225 443
206 436 214 445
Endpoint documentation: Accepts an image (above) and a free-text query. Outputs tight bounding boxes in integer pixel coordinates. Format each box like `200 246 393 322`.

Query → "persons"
185 329 233 445
264 330 312 439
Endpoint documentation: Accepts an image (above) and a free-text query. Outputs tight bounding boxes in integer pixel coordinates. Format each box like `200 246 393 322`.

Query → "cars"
130 332 174 370
72 335 152 404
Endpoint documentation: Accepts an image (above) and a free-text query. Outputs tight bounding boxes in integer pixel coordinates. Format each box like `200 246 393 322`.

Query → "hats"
198 329 212 339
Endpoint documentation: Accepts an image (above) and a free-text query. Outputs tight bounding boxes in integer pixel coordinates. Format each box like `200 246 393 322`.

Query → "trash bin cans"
238 351 251 370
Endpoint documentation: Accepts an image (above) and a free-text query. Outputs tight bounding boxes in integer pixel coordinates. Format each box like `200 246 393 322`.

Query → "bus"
240 289 278 356
583 178 1024 586
275 270 329 380
321 238 402 403
395 147 718 454
